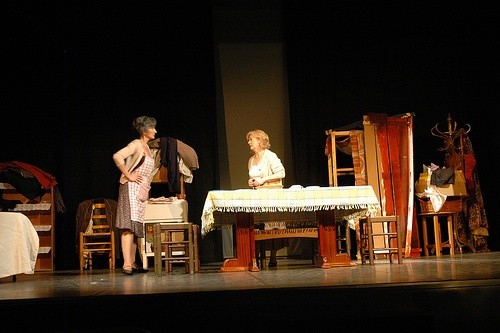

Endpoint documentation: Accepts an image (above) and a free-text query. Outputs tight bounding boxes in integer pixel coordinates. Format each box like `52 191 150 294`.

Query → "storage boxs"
415 169 469 212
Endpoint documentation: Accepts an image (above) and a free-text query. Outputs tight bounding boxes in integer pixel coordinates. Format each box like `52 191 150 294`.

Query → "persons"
113 117 160 275
246 130 285 266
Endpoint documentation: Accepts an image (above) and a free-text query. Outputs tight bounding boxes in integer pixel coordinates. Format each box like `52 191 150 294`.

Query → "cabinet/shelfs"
0 182 54 272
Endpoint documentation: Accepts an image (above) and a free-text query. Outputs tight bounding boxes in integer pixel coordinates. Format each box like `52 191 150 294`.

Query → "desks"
416 212 460 257
0 212 40 282
201 185 379 272
135 198 189 269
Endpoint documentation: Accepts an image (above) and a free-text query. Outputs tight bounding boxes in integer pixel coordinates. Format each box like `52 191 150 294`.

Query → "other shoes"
268 261 277 267
121 264 149 275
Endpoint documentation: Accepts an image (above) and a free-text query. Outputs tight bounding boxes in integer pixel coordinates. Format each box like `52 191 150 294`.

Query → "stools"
336 214 403 267
152 222 201 272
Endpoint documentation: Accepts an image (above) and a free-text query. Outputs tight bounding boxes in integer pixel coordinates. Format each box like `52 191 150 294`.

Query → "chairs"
77 199 119 270
